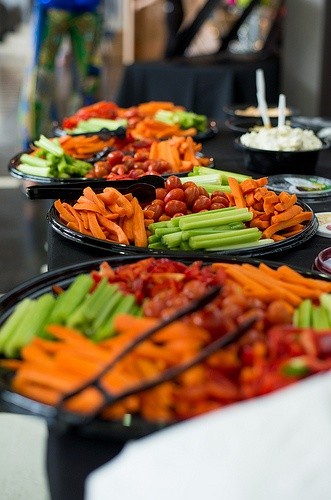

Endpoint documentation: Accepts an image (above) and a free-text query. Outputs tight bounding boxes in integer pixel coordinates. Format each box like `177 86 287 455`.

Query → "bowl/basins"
222 104 300 126
234 129 328 176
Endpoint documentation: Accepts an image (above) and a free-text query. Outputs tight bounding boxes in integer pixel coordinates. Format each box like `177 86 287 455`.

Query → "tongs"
25 175 167 202
46 283 260 438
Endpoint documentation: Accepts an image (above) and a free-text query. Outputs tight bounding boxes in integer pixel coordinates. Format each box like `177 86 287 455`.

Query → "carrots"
150 135 210 171
213 262 331 313
53 186 148 247
228 177 314 240
0 316 208 420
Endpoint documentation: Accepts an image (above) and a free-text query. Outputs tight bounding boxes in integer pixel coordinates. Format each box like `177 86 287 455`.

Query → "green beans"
86 150 171 181
142 280 297 333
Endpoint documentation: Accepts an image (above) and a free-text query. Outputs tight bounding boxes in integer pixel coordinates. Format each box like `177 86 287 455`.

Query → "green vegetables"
17 134 93 179
180 165 252 197
1 274 142 356
147 206 275 251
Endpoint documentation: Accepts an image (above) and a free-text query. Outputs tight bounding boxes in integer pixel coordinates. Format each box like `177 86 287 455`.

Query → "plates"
9 140 104 183
260 175 331 201
41 174 320 264
2 253 330 439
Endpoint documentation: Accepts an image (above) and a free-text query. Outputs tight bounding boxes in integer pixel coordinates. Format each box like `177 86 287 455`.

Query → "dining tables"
0 116 331 499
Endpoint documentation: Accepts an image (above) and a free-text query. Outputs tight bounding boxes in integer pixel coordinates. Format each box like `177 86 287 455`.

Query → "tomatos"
144 175 230 221
